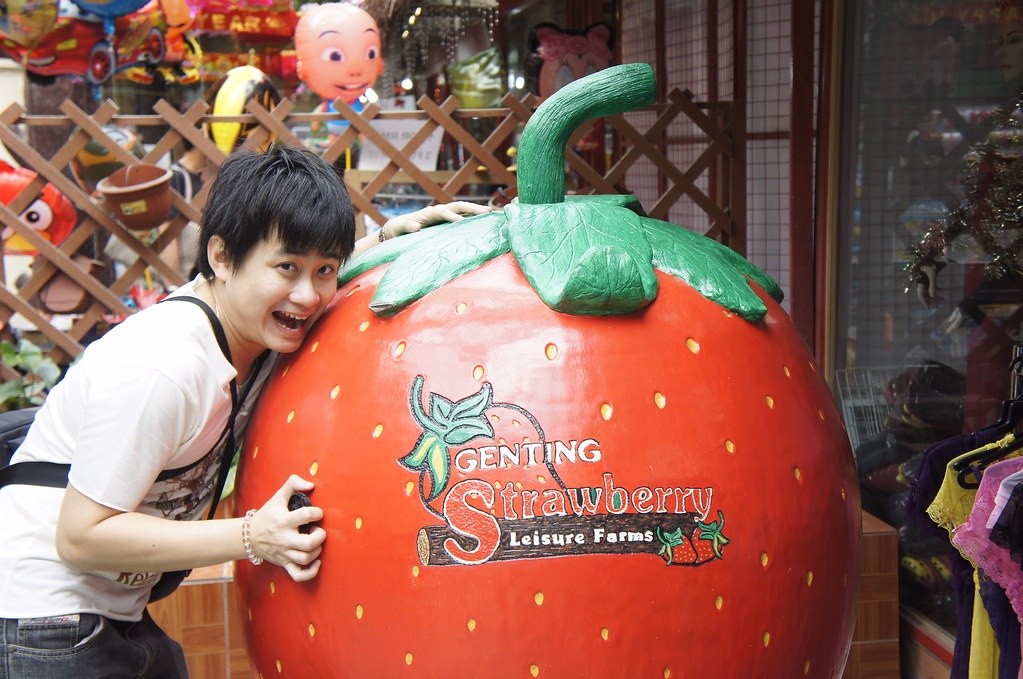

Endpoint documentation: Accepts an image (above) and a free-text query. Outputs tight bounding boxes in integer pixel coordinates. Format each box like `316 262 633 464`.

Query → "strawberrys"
224 62 858 679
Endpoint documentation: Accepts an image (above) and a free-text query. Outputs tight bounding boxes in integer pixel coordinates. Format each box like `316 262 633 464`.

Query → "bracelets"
242 508 264 565
378 219 390 242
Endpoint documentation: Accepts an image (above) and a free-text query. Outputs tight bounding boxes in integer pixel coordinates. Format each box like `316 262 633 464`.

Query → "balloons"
203 64 282 156
296 3 383 142
0 0 203 86
523 22 617 104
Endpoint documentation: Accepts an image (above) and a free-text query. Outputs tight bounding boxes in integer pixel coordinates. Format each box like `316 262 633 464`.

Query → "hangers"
920 356 1023 491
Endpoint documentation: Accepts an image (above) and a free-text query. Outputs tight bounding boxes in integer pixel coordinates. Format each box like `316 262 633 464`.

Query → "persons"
0 143 493 679
